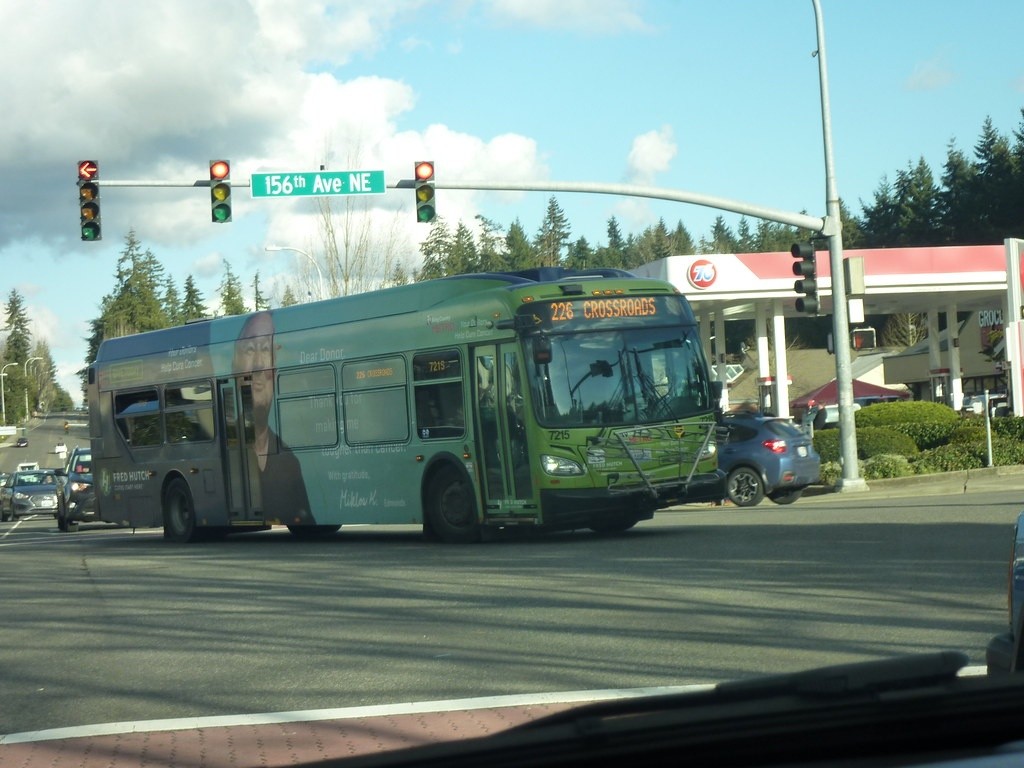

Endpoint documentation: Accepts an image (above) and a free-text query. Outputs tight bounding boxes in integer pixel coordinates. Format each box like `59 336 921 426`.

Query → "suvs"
56 444 93 530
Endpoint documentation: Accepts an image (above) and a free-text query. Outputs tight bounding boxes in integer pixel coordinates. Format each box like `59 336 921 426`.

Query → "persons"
217 313 307 526
43 474 54 486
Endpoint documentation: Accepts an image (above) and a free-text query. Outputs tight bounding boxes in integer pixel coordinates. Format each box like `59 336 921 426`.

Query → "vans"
17 462 41 482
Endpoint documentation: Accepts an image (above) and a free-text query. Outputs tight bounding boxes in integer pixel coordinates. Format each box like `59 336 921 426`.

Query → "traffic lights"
77 159 105 242
414 160 438 224
851 326 878 351
209 158 234 223
792 241 822 315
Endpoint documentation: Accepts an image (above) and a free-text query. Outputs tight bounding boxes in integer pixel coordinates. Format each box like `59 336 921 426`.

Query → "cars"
0 472 12 492
714 408 822 508
0 469 62 523
54 442 68 454
17 438 29 447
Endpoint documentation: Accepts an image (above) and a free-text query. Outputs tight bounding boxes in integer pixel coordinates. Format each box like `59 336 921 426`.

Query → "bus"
88 265 730 546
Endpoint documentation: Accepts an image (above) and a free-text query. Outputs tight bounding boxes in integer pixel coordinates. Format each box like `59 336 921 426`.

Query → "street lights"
24 356 47 422
1 362 19 424
266 245 323 301
